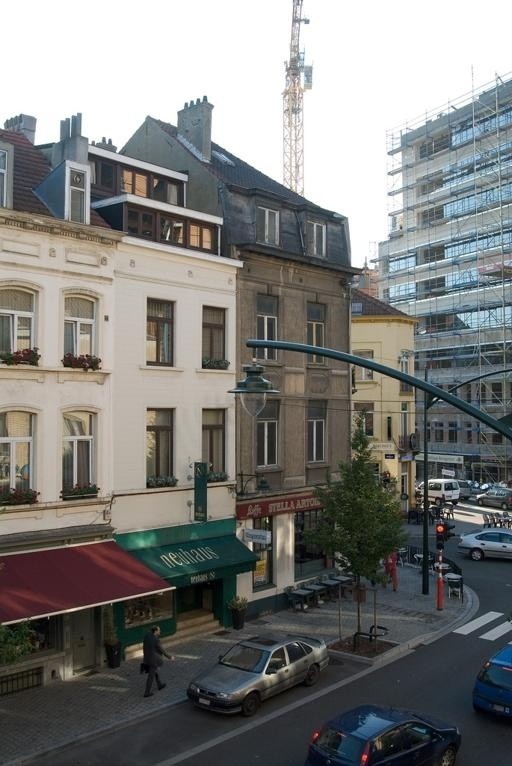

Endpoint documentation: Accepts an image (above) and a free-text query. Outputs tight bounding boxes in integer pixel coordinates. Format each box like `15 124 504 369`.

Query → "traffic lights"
436 521 456 550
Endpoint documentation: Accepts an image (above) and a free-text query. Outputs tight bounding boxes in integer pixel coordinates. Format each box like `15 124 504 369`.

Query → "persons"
381 548 399 591
143 625 176 697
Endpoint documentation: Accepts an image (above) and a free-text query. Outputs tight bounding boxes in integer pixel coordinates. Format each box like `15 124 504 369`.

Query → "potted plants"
208 472 229 483
204 357 230 370
145 474 177 488
226 592 248 630
104 604 121 669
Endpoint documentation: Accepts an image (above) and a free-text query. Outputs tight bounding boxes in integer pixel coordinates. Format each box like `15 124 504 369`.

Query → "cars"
415 479 512 510
304 643 512 766
456 528 512 561
187 631 330 717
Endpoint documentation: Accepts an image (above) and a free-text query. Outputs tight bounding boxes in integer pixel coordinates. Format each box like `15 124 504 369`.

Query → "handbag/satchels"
140 663 151 675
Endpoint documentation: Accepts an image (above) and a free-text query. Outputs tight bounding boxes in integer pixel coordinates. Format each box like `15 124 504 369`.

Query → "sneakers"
144 692 154 697
157 682 166 690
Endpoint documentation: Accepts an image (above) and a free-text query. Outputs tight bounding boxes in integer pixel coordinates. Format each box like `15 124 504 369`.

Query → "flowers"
62 353 101 371
0 486 37 505
1 346 40 367
59 482 100 498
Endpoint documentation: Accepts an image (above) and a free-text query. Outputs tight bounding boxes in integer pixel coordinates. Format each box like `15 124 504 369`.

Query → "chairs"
284 572 344 612
398 547 462 599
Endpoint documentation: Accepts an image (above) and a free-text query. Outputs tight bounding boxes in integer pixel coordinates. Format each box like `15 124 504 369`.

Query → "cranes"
282 0 314 198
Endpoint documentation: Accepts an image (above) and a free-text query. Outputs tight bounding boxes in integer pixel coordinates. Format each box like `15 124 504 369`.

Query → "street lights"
227 339 512 441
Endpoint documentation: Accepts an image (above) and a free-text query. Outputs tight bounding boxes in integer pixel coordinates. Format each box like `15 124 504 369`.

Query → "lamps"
235 470 272 495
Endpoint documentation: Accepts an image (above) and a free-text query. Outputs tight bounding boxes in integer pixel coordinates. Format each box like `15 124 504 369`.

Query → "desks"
333 576 353 598
